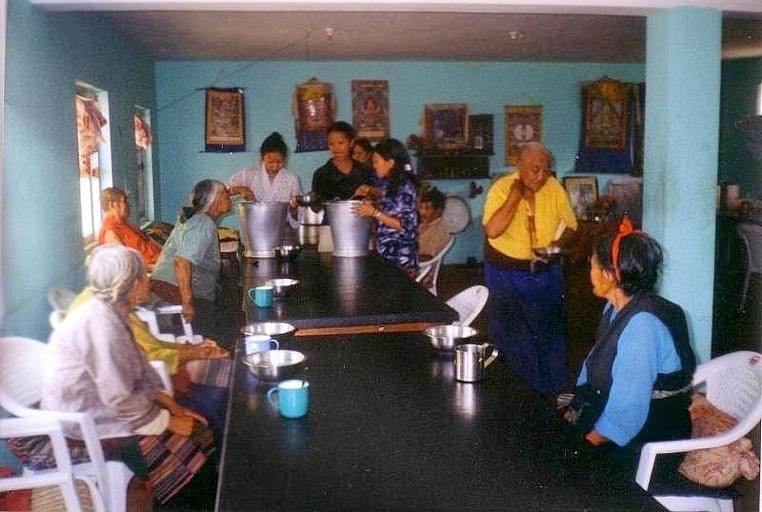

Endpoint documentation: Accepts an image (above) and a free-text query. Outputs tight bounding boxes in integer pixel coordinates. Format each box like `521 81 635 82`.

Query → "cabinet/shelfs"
412 138 491 180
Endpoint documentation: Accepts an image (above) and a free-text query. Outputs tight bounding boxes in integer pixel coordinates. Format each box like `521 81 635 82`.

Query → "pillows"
675 388 762 492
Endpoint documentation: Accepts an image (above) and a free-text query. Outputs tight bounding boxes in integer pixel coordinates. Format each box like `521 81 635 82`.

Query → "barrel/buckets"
233 200 289 259
322 200 375 259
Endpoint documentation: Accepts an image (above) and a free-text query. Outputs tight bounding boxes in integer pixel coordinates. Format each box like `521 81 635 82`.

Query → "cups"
451 343 501 383
268 379 310 418
245 336 279 354
739 201 751 215
248 286 274 308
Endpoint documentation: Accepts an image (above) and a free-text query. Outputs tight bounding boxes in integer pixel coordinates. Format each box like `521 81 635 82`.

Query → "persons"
564 232 695 488
481 142 578 396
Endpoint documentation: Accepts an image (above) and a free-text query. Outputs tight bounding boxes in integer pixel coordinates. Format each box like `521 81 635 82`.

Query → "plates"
442 195 472 235
531 247 565 259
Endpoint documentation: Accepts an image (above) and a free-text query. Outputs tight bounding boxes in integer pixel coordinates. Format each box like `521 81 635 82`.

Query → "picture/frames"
425 103 469 147
562 174 601 218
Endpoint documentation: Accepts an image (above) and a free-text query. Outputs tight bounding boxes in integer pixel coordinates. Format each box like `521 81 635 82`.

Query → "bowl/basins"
295 194 317 207
241 351 306 381
257 277 298 298
273 245 304 262
240 322 298 345
423 323 479 349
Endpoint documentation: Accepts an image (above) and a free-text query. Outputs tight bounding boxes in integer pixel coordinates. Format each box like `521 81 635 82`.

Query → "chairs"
632 347 761 511
444 281 492 324
733 216 762 314
0 337 178 512
1 418 106 512
415 231 457 298
45 274 205 414
